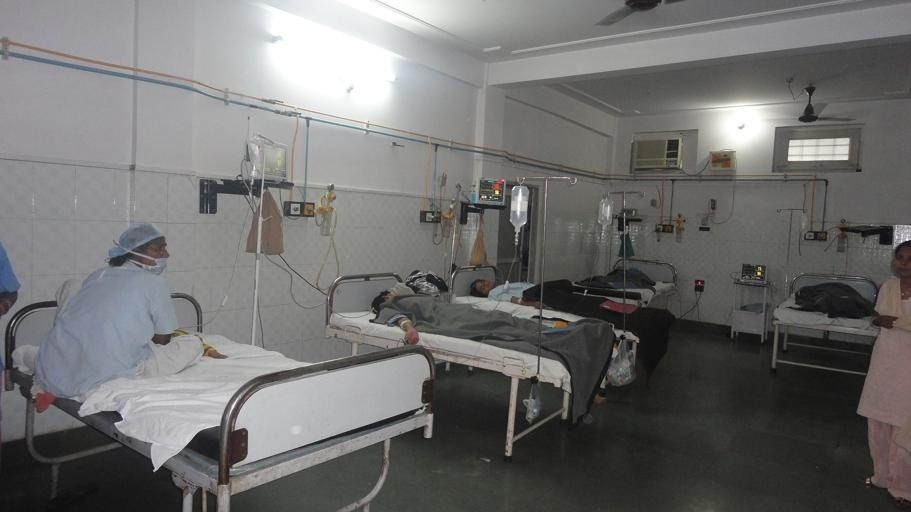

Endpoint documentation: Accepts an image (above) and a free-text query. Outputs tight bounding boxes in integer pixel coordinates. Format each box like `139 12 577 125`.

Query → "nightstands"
729 280 773 344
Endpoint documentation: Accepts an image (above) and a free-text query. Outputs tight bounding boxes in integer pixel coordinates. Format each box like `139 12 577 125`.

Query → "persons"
471 279 547 310
0 242 23 393
34 221 205 415
855 241 910 505
370 290 420 344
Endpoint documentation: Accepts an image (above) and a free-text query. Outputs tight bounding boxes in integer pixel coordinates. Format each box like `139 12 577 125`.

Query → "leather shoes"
894 495 911 511
858 474 885 489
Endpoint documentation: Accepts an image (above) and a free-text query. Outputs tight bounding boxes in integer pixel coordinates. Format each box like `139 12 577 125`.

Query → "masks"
133 252 169 276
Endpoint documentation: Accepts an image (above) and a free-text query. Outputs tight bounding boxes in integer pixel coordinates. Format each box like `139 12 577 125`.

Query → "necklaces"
901 286 910 299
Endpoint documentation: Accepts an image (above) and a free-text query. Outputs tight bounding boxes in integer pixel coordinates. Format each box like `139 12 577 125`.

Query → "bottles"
443 213 450 238
249 143 263 178
320 201 333 238
799 214 808 238
508 184 533 235
598 198 613 230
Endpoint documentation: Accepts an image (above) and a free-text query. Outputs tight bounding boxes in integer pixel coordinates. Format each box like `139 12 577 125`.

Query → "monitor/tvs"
475 179 506 206
741 263 767 284
242 139 289 181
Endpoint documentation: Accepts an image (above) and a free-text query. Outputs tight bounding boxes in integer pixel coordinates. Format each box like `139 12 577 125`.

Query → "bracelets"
519 298 523 303
2 298 14 311
400 320 413 329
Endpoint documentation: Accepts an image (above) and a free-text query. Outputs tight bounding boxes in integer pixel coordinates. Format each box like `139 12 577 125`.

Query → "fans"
592 0 683 27
764 85 856 122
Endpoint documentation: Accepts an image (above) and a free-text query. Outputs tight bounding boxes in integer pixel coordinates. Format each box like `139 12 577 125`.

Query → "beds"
450 266 675 405
570 257 678 311
323 271 614 464
770 274 880 376
4 293 436 512
